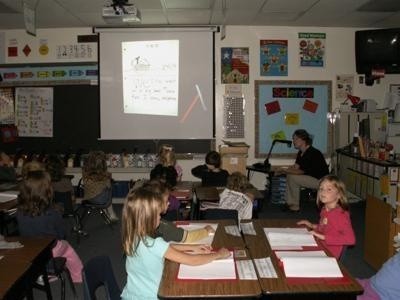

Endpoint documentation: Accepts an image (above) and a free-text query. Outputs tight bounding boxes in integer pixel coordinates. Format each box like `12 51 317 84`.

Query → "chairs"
74 180 115 233
82 256 122 300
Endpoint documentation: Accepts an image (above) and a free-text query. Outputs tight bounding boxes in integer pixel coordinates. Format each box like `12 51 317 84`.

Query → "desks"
157 161 364 300
0 179 57 300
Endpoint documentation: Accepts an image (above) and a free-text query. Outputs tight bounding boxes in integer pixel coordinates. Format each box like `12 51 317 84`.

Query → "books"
171 222 350 285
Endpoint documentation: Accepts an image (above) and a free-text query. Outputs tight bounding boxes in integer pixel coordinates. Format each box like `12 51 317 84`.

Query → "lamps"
341 94 361 106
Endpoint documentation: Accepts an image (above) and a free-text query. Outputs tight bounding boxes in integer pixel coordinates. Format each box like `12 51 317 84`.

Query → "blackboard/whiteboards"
0 78 216 160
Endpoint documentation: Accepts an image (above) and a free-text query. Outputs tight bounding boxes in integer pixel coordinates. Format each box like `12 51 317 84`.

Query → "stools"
24 257 78 300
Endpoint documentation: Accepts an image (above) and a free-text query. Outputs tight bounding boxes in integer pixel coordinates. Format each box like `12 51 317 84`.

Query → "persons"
0 144 255 300
277 129 329 214
296 175 356 260
354 250 400 300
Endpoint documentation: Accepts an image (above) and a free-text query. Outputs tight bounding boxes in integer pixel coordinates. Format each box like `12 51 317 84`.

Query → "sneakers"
280 202 300 213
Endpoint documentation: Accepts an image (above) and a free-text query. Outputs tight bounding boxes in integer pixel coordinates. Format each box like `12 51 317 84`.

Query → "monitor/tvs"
354 28 400 73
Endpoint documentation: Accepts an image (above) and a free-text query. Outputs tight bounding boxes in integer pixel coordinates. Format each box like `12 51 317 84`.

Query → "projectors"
102 5 141 23
